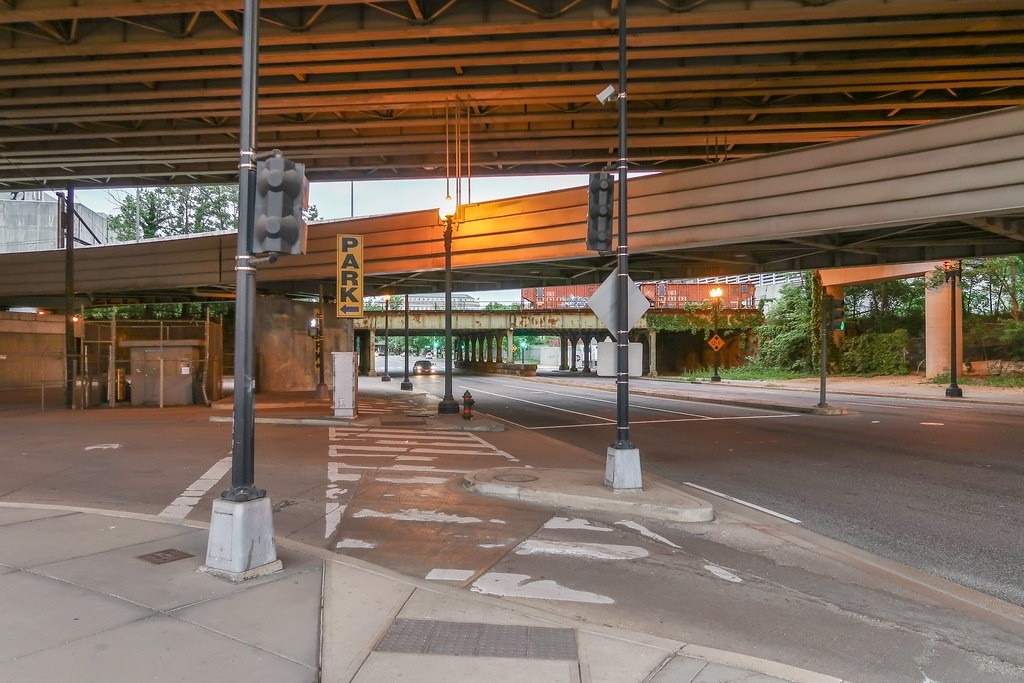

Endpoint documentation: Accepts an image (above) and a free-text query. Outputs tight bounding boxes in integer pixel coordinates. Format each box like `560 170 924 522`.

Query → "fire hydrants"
463 390 475 420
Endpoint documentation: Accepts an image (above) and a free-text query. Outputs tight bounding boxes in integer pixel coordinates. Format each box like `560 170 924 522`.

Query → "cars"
413 360 432 374
426 353 432 359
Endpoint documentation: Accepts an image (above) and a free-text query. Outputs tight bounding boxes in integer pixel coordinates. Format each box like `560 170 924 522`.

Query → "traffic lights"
830 295 847 333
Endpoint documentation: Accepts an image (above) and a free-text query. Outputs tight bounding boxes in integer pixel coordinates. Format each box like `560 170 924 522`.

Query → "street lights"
709 287 725 382
437 194 464 415
381 295 393 382
